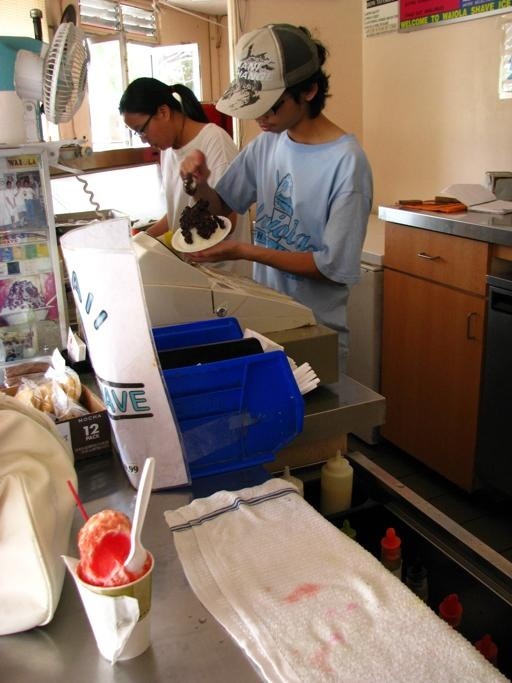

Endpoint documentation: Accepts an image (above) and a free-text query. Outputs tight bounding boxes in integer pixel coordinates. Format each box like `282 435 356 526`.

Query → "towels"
163 474 511 678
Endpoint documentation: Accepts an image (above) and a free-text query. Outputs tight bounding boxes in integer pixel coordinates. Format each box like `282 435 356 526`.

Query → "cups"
75 548 155 661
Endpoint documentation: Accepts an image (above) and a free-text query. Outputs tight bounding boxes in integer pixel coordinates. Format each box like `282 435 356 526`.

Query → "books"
399 184 512 216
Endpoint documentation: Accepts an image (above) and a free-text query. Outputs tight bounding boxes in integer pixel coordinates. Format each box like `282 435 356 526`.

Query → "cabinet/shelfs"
380 202 487 494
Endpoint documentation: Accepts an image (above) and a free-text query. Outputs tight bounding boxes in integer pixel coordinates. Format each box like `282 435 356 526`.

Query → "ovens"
469 271 512 516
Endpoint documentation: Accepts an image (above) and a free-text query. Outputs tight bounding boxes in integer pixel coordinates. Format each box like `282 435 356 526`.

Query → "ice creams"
1 279 54 324
73 508 153 667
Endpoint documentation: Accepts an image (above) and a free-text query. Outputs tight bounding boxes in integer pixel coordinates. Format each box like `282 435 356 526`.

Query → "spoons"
123 453 158 570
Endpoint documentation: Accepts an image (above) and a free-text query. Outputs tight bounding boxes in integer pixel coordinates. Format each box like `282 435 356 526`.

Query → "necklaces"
177 116 186 143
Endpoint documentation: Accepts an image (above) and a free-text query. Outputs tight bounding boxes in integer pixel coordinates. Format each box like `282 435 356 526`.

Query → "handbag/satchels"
1 391 78 635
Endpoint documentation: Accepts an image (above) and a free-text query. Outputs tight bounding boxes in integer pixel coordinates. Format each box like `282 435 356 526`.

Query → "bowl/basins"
1 305 54 327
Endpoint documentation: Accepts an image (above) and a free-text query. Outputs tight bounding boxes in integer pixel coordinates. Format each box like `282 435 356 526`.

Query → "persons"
117 77 253 276
179 24 374 366
0 175 40 229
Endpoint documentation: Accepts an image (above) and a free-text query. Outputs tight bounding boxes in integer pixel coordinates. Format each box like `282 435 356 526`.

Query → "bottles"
321 448 354 515
279 464 305 501
339 519 501 668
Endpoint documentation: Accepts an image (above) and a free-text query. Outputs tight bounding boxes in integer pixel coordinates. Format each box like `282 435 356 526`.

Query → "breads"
14 368 86 422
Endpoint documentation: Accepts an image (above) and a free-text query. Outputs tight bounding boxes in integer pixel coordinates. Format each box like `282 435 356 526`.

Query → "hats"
216 24 321 121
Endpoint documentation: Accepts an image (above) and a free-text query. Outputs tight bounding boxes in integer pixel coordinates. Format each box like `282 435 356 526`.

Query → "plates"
171 214 231 253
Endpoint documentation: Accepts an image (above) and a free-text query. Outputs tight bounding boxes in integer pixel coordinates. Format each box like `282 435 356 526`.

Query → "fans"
9 22 91 177
